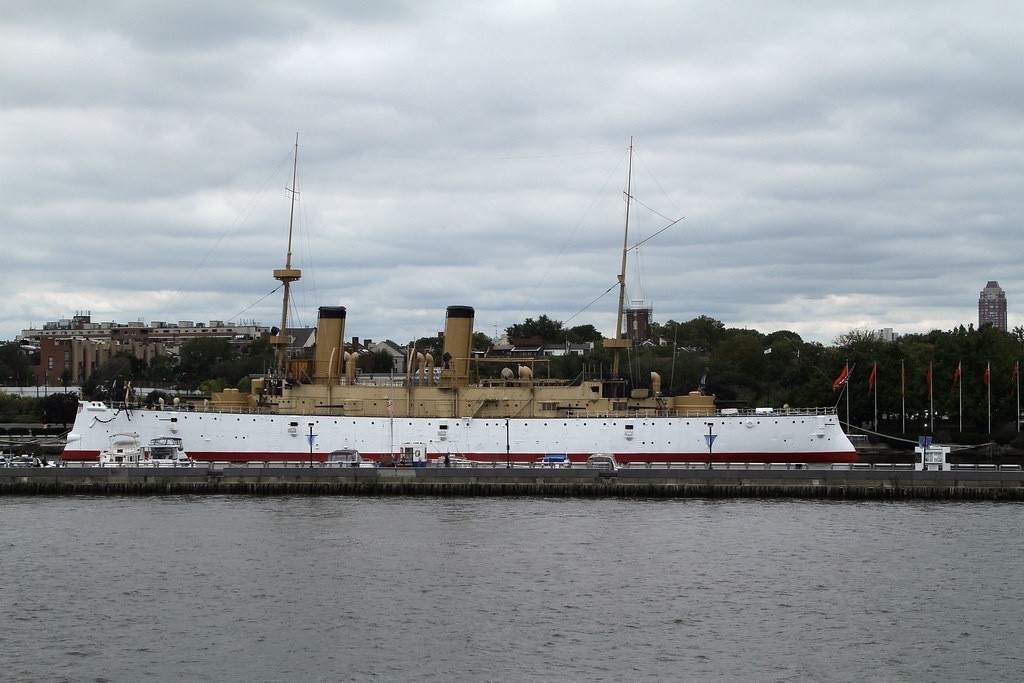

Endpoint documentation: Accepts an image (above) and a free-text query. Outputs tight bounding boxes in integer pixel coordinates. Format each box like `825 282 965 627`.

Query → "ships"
57 123 872 470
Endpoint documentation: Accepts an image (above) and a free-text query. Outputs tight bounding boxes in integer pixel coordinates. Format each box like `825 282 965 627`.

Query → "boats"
96 431 143 468
0 448 44 468
848 435 1024 471
143 436 193 469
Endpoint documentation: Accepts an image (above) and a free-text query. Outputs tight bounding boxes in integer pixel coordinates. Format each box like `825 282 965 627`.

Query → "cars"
542 453 571 470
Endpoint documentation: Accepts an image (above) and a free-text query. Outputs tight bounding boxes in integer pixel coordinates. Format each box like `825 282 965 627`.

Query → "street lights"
707 422 714 470
921 419 930 471
504 415 513 469
308 422 315 469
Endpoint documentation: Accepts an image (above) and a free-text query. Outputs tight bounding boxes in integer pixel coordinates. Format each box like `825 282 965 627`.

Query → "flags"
950 365 961 394
926 363 933 401
1009 363 1019 398
984 368 990 400
832 363 848 392
867 365 876 400
